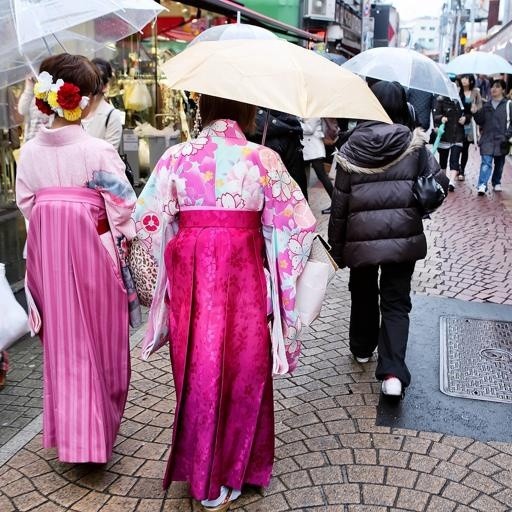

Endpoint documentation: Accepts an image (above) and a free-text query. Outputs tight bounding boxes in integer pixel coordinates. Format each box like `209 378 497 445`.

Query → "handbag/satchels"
468 122 481 142
412 172 447 221
295 259 329 326
128 240 159 308
309 231 339 288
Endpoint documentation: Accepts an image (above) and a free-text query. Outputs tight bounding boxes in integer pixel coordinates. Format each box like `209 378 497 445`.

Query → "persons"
407 73 512 195
81 57 123 151
129 92 316 511
326 79 449 397
248 106 349 213
17 75 57 263
15 52 136 464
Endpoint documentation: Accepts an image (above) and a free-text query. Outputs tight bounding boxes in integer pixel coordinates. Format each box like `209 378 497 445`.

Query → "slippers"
204 486 233 512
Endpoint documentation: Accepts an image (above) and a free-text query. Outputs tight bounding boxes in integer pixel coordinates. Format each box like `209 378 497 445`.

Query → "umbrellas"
159 39 395 128
0 1 172 91
186 22 279 50
1 32 118 132
443 51 512 74
339 47 458 99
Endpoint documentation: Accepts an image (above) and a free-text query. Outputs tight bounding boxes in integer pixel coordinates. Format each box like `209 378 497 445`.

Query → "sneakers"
448 174 503 195
382 374 403 396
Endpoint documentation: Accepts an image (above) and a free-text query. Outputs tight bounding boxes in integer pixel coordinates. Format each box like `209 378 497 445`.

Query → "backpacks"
321 118 340 146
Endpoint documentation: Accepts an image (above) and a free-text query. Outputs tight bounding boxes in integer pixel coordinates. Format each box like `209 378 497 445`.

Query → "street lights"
459 32 468 55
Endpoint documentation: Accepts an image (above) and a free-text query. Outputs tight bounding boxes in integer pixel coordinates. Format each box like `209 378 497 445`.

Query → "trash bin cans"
120 128 139 187
148 136 181 175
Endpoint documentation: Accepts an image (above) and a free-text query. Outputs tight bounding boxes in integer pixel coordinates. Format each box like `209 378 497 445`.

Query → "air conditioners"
301 0 337 19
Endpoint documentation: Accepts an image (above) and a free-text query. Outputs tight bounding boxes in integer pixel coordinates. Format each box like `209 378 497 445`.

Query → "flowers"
29 71 91 121
